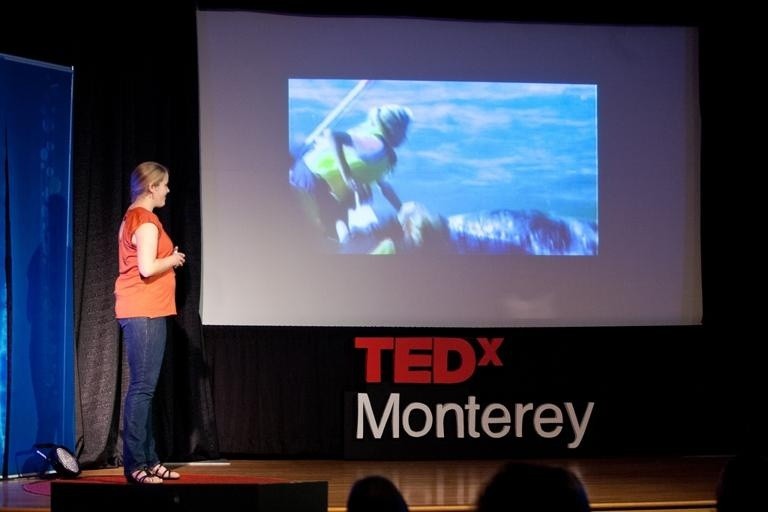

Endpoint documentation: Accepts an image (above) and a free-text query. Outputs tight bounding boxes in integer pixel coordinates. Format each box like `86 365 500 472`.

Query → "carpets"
20 473 283 497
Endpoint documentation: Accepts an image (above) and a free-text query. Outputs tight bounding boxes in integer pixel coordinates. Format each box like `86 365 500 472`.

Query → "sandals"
126 469 163 484
145 462 179 480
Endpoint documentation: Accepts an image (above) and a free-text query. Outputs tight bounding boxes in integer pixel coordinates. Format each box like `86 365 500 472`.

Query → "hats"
369 102 411 148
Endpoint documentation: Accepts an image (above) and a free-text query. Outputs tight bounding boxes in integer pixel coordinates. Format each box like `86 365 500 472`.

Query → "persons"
289 103 462 256
471 457 593 511
345 474 411 511
714 444 767 512
112 160 185 484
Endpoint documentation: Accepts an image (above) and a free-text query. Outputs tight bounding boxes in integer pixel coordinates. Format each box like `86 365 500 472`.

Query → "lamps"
34 443 81 480
